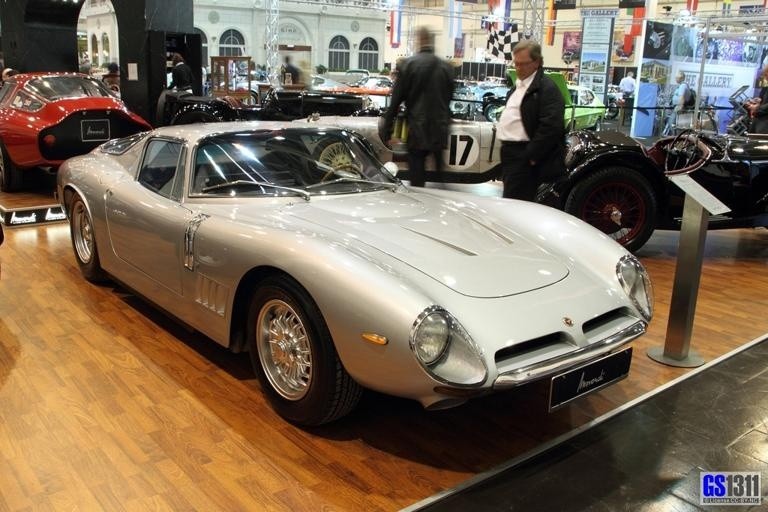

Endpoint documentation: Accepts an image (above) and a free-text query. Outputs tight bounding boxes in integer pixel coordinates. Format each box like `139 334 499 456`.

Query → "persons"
380 27 454 185
283 56 302 84
1 67 19 85
102 63 121 84
168 53 196 96
496 39 565 197
618 71 636 106
668 72 691 135
749 66 768 134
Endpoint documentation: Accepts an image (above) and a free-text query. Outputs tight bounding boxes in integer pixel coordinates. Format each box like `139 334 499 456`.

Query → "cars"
449 69 623 132
156 90 365 126
310 69 393 96
197 62 270 110
281 97 501 183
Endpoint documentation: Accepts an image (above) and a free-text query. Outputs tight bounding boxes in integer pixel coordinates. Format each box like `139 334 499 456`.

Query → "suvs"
534 103 768 253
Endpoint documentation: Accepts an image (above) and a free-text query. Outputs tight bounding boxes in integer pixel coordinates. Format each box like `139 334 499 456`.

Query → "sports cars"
57 120 654 427
0 68 154 191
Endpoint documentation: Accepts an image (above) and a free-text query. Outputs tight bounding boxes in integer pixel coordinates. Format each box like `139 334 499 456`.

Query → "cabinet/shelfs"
207 55 252 106
